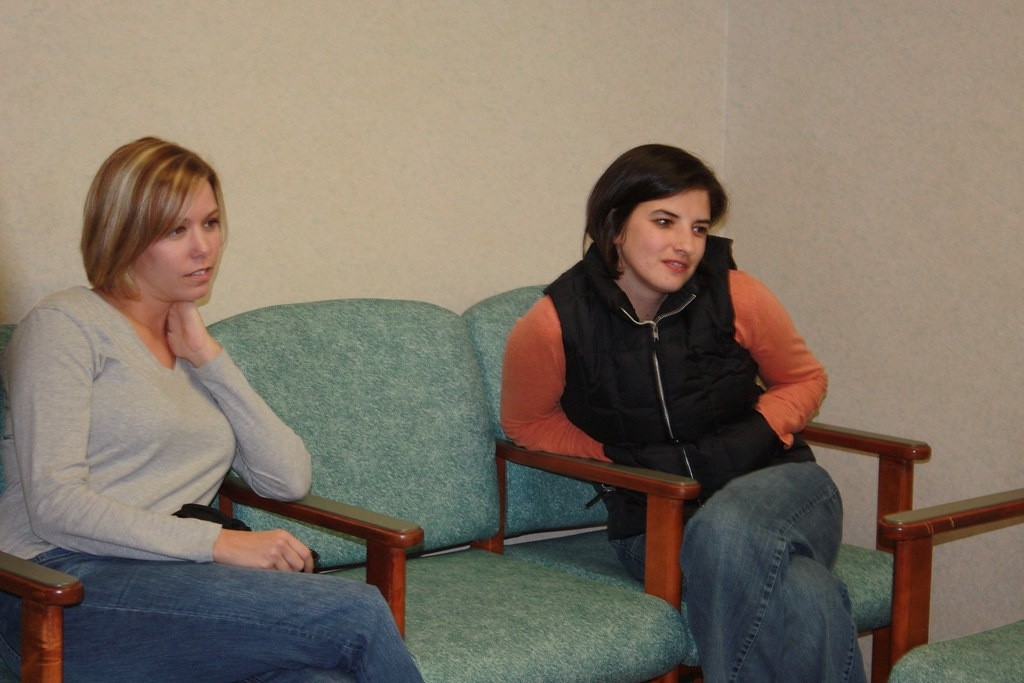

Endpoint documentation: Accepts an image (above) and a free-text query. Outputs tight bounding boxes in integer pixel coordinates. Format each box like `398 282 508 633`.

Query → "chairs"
0 283 931 683
877 485 1024 683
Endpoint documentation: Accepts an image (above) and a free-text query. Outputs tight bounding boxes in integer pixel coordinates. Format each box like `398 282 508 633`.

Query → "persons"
0 137 426 683
499 145 867 683
